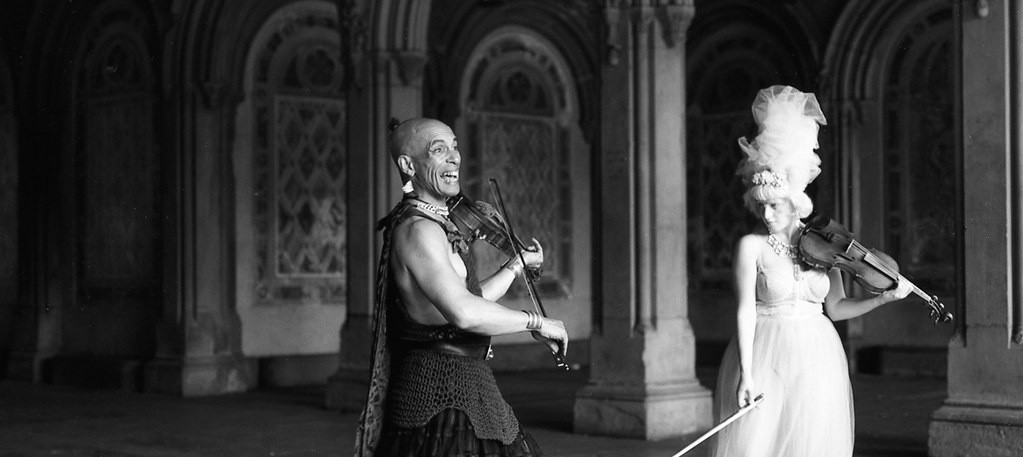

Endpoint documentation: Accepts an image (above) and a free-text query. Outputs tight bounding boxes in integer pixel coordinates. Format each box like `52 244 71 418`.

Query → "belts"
392 340 496 361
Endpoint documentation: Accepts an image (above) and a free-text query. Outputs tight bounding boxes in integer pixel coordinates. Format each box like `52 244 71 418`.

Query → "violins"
796 214 954 327
446 187 545 283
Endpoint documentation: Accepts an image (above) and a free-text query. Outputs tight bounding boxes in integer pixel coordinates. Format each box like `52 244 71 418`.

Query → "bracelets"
521 310 543 332
500 258 523 280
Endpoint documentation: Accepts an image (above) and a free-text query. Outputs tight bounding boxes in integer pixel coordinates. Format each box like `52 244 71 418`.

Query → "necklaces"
767 232 803 280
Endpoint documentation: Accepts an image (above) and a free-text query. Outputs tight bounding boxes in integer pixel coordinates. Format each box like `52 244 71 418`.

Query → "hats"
734 83 828 186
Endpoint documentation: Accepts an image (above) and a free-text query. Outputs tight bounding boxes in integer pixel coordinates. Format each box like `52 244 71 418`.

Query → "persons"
709 84 914 457
353 117 568 456
